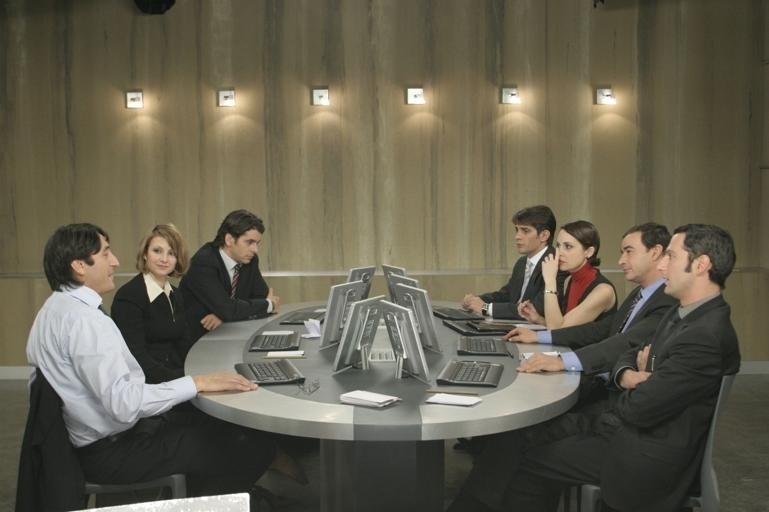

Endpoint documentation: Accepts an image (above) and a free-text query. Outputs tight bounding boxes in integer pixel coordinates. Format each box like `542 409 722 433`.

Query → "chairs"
576 370 735 511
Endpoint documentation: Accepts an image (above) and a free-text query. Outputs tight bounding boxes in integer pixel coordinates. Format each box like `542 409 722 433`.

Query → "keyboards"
279 311 326 324
250 330 301 351
438 359 502 387
457 337 510 355
442 320 507 335
235 359 305 387
432 307 484 320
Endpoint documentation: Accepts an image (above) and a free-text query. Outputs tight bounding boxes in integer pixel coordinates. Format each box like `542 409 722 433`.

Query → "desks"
182 298 583 511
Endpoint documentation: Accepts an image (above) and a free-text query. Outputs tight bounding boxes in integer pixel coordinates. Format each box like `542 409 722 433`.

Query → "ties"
521 257 534 302
616 290 644 336
230 265 241 300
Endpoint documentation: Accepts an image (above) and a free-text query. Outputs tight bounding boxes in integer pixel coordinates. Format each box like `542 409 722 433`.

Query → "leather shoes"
451 435 482 456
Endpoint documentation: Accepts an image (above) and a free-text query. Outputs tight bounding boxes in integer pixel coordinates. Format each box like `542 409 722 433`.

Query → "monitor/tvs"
318 264 439 387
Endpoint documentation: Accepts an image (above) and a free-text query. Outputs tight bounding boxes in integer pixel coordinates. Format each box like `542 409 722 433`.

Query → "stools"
34 363 188 509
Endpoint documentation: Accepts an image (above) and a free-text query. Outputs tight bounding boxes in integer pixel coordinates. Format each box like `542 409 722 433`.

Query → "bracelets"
543 290 560 295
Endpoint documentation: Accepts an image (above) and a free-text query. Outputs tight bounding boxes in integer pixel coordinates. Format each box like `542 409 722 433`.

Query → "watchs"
482 303 490 315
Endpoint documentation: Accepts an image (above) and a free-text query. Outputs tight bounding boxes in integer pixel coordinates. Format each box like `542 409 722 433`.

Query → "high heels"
252 486 302 511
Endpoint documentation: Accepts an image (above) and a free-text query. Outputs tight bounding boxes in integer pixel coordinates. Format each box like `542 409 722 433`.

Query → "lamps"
501 87 520 104
126 91 144 109
596 87 616 105
406 87 426 106
217 89 235 108
312 88 331 107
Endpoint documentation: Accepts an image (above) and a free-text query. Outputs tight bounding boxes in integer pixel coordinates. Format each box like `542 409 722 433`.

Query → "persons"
113 222 209 381
443 223 741 512
517 220 619 335
502 221 673 411
462 205 569 317
25 221 249 495
178 210 281 345
